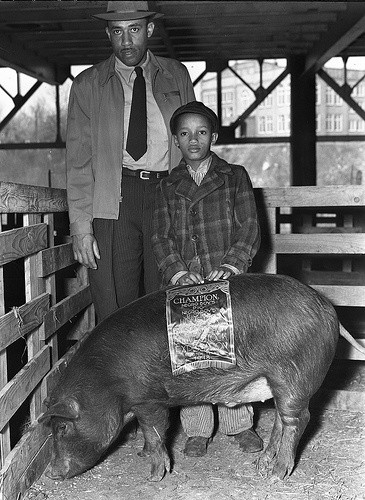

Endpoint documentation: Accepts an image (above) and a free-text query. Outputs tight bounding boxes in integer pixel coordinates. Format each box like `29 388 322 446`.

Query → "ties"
125 66 147 161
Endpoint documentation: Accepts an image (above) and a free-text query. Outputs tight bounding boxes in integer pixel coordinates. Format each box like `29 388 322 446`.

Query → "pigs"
37 270 365 485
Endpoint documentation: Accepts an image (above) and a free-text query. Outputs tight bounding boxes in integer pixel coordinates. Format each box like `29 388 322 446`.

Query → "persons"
151 101 264 457
65 0 196 324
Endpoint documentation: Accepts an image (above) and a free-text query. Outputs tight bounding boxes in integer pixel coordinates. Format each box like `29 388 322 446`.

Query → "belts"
122 165 169 179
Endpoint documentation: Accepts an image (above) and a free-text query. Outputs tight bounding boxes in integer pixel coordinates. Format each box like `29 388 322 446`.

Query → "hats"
93 0 165 21
169 100 219 132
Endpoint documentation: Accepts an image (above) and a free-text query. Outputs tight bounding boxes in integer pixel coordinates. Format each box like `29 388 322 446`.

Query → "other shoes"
233 426 264 453
182 436 208 456
